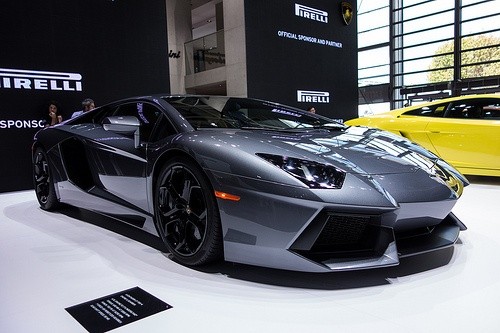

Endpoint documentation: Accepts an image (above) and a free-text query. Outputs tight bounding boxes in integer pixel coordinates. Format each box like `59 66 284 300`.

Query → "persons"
306 106 315 114
71 98 96 119
42 103 63 128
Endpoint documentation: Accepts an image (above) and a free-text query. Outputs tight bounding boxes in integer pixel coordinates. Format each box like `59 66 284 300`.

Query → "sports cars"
29 95 471 274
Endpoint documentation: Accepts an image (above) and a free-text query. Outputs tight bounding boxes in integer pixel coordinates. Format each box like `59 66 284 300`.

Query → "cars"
343 93 500 177
